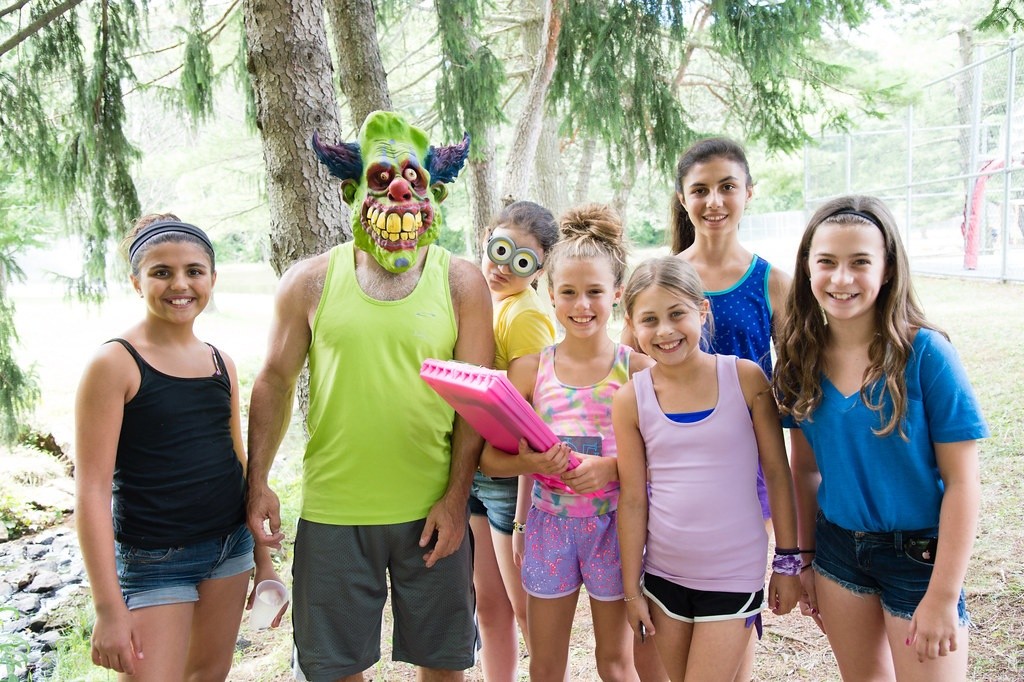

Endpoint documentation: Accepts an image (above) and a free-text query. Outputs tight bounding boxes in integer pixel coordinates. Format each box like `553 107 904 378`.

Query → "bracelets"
512 519 526 534
624 589 643 602
798 563 812 574
799 548 816 554
772 547 799 576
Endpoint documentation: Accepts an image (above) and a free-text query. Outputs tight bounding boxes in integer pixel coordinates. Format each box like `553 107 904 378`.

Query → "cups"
248 580 287 630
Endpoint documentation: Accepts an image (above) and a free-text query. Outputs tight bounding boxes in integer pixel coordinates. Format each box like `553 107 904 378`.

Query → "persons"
621 138 796 682
466 200 562 682
611 256 804 680
247 110 495 682
74 215 289 682
772 194 987 682
479 207 656 680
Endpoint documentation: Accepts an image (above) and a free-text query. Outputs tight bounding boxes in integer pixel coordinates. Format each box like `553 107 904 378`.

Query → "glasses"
488 235 544 278
